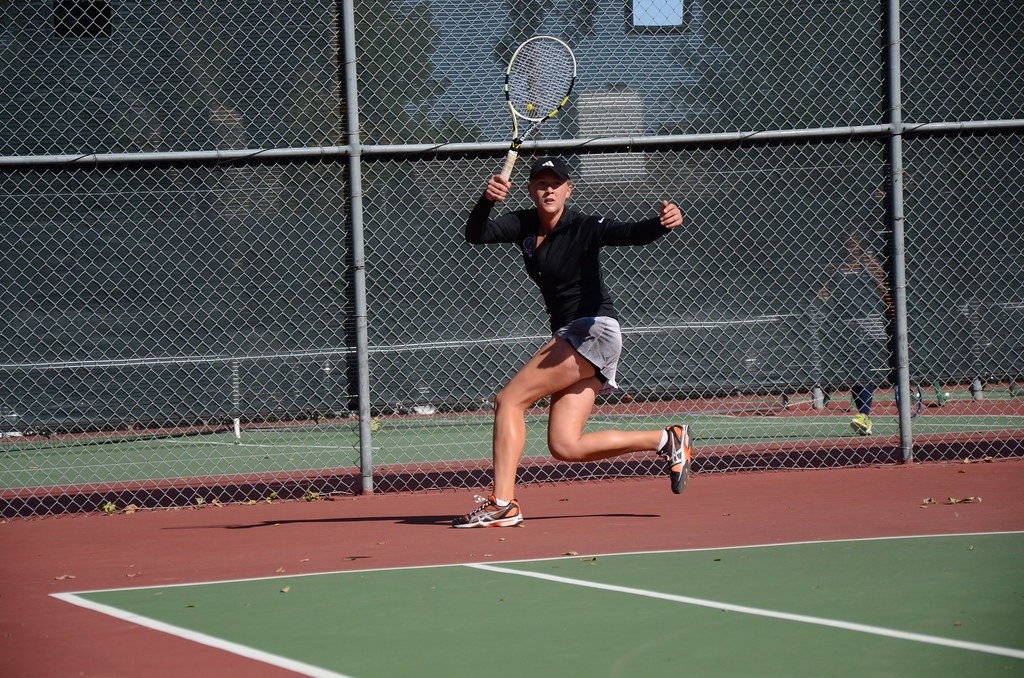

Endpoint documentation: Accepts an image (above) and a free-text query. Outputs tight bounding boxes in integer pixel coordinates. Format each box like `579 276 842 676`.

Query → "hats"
529 157 571 182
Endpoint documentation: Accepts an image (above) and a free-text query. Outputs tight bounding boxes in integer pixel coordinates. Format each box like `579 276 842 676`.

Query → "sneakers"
654 424 693 494
371 416 378 431
452 494 524 529
850 415 871 431
859 419 872 436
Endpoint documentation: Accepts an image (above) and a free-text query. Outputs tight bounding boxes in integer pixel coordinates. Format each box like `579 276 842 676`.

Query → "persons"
450 159 691 530
814 225 895 436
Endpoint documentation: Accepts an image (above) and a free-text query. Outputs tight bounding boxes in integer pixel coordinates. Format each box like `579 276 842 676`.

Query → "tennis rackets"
500 35 577 180
